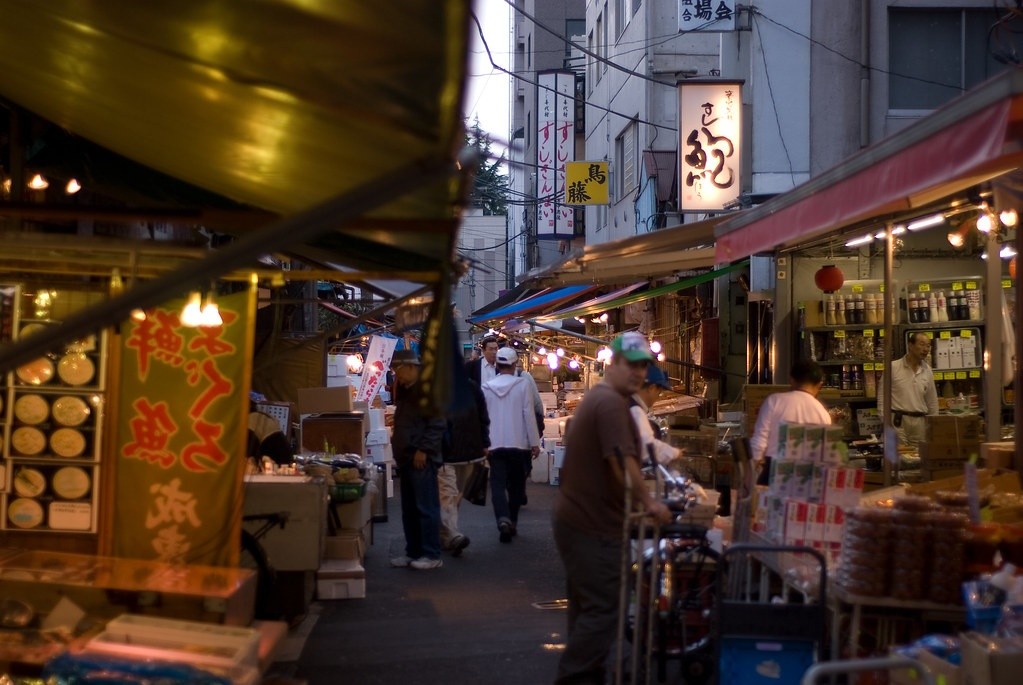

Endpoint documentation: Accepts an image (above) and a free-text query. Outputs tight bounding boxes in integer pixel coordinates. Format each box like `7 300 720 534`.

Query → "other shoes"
450 535 470 558
498 520 509 544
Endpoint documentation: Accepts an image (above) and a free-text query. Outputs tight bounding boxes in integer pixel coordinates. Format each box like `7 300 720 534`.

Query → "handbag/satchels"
464 458 490 507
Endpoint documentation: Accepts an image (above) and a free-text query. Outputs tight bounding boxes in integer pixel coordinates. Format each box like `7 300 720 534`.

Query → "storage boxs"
352 401 394 498
749 421 865 591
637 479 734 540
889 605 1023 685
934 335 976 369
297 355 352 413
0 474 379 628
918 415 981 480
530 418 565 486
855 408 881 436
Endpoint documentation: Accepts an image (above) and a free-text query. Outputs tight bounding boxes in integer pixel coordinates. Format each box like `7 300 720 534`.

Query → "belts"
892 410 925 417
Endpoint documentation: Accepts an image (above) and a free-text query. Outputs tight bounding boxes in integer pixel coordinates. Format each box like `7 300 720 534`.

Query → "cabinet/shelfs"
805 318 902 484
900 320 987 420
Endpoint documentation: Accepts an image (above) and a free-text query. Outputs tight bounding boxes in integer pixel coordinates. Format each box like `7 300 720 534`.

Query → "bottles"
821 364 862 390
326 506 342 531
855 294 865 323
837 295 846 324
981 575 991 593
878 294 885 324
980 564 1015 607
918 293 929 322
947 292 958 320
935 382 979 415
892 295 896 323
909 292 919 323
937 292 948 322
847 295 855 324
866 294 877 324
292 463 297 475
928 293 939 321
957 290 969 320
827 296 836 325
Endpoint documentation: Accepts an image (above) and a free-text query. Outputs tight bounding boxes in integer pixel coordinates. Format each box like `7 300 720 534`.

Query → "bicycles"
622 442 730 685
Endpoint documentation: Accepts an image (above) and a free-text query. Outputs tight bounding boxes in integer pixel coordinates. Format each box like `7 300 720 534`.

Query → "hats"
495 346 518 364
610 332 655 362
389 349 420 365
644 364 673 388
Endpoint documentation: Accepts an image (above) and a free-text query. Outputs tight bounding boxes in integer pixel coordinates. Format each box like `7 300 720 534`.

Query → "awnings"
716 66 1023 257
466 206 742 326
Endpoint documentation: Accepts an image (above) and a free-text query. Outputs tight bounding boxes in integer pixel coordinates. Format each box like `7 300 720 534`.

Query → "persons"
243 396 295 467
750 360 834 487
390 336 544 571
876 334 938 460
552 330 687 685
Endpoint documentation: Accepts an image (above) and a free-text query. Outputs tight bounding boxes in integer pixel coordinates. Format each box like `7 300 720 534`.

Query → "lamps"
180 277 223 328
948 216 976 247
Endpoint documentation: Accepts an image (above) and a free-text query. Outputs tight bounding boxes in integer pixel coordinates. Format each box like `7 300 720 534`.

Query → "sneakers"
412 557 443 568
393 557 412 565
